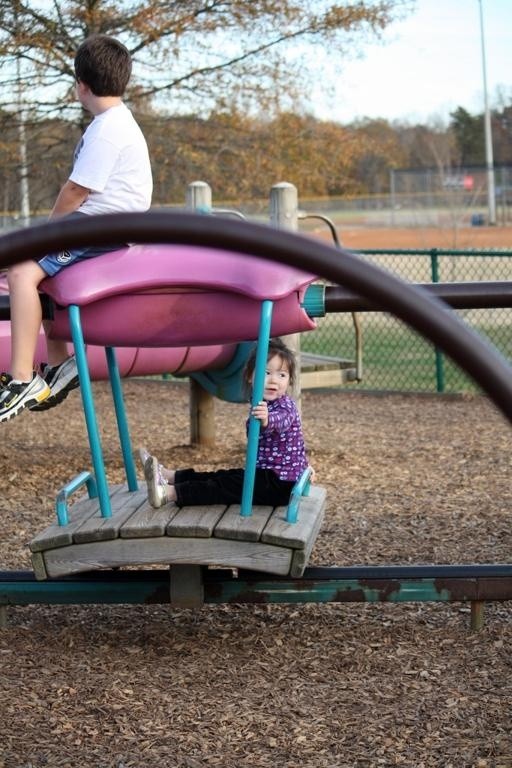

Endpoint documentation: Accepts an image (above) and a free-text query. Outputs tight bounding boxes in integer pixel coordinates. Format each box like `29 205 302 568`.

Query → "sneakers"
145 456 167 508
0 371 51 423
28 354 80 411
138 448 169 484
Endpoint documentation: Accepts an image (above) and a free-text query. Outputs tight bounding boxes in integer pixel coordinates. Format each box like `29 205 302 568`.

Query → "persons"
133 339 308 509
0 34 154 428
